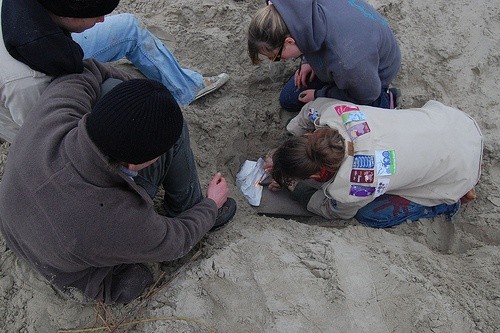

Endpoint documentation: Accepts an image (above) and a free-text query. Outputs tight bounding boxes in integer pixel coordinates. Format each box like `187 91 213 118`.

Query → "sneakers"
194 72 230 100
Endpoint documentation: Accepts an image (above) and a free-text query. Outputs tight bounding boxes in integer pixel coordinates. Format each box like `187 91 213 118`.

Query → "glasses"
271 38 285 62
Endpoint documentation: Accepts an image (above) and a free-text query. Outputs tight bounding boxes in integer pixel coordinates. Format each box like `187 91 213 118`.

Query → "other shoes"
461 187 475 206
388 87 401 110
209 197 236 232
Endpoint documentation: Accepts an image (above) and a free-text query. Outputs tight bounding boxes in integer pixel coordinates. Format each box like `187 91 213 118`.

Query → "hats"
87 79 184 165
40 0 121 19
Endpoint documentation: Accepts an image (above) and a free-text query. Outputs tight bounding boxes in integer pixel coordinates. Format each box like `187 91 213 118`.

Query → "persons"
0 57 237 304
264 97 484 227
0 0 229 144
247 0 403 115
